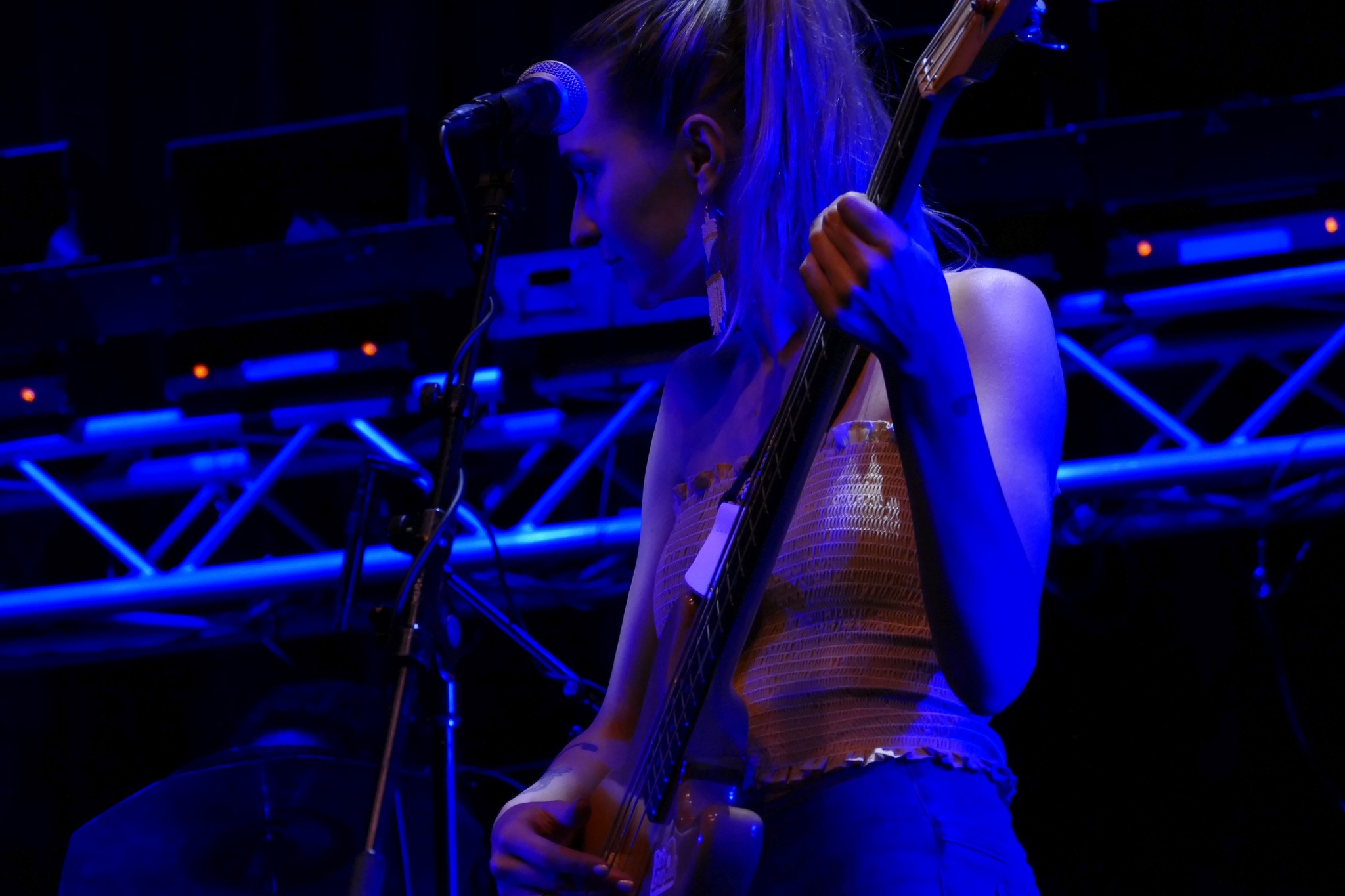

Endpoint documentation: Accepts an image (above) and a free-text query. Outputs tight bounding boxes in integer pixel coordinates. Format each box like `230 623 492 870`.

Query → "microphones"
443 60 589 140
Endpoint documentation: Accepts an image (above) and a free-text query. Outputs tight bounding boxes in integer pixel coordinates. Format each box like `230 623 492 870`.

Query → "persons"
489 0 1065 896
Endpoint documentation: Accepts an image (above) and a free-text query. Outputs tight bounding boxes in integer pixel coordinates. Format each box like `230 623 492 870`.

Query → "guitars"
558 0 1069 893
